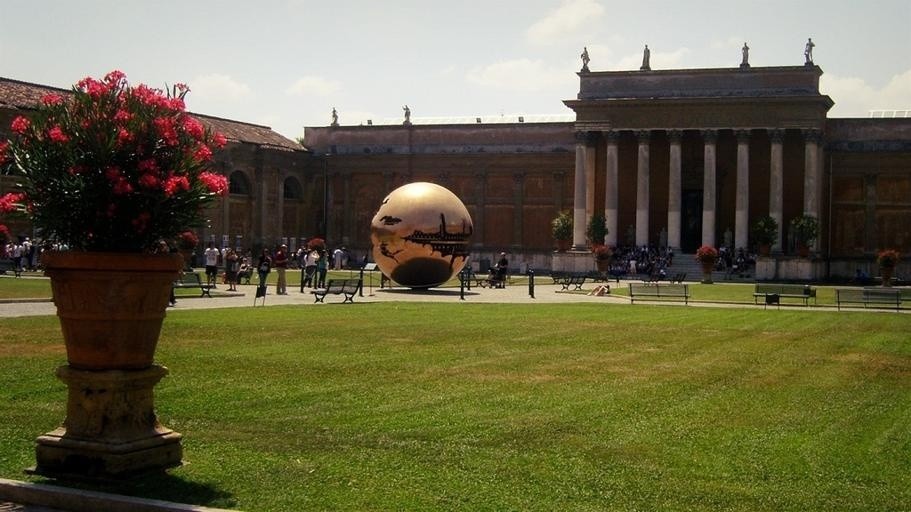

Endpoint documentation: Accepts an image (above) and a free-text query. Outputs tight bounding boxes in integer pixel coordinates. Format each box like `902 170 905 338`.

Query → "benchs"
457 268 490 288
550 270 586 292
173 272 218 298
751 282 903 314
310 278 363 304
629 282 691 305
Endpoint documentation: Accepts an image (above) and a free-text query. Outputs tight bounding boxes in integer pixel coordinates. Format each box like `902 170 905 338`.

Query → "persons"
740 41 750 65
658 226 668 247
804 37 815 62
190 241 350 295
331 107 338 124
606 244 673 281
722 227 732 248
0 234 70 276
495 251 508 288
851 268 866 285
623 223 635 245
485 266 502 288
640 44 651 68
401 103 410 121
579 46 590 67
155 240 176 306
711 243 759 281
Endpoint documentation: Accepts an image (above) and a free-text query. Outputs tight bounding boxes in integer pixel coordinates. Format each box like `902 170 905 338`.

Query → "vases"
35 251 184 372
702 262 714 273
880 267 892 277
597 260 609 271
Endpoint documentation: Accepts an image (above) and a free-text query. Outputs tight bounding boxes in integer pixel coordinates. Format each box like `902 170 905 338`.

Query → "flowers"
591 244 613 259
693 245 718 264
0 70 231 255
878 250 901 267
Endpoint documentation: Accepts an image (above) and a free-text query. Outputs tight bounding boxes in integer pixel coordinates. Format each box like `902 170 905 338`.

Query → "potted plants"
551 214 571 251
751 215 779 257
584 215 609 246
791 213 822 257
